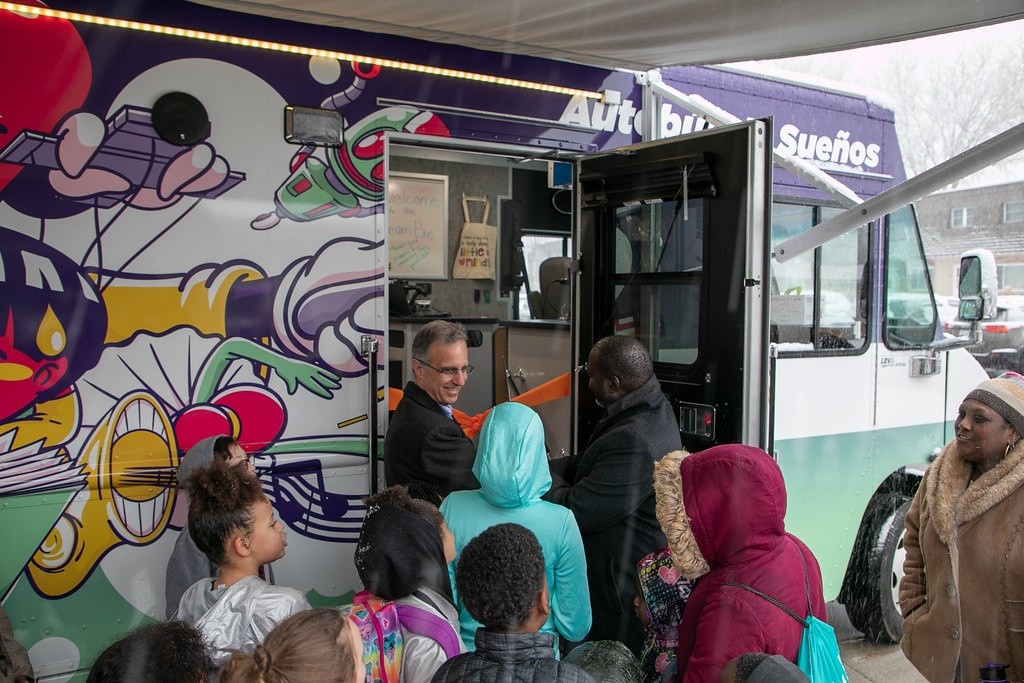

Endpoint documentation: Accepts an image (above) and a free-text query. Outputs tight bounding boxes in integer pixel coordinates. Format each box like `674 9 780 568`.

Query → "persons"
176 458 314 683
165 433 277 631
438 399 593 669
221 607 367 683
652 444 828 683
355 484 468 683
384 319 487 513
630 542 697 683
85 617 209 683
536 334 686 667
719 652 812 683
430 522 604 683
898 369 1024 683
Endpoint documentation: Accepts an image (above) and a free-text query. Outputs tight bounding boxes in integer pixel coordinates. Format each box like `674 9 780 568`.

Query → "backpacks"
334 592 459 683
723 541 849 683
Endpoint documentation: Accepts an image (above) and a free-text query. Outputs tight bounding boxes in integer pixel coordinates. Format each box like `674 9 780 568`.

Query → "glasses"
231 454 255 472
414 358 475 376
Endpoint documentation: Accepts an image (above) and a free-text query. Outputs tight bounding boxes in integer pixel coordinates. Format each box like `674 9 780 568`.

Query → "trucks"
0 1 1022 683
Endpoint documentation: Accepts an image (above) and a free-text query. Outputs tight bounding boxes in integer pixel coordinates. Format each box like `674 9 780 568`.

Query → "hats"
963 372 1023 436
